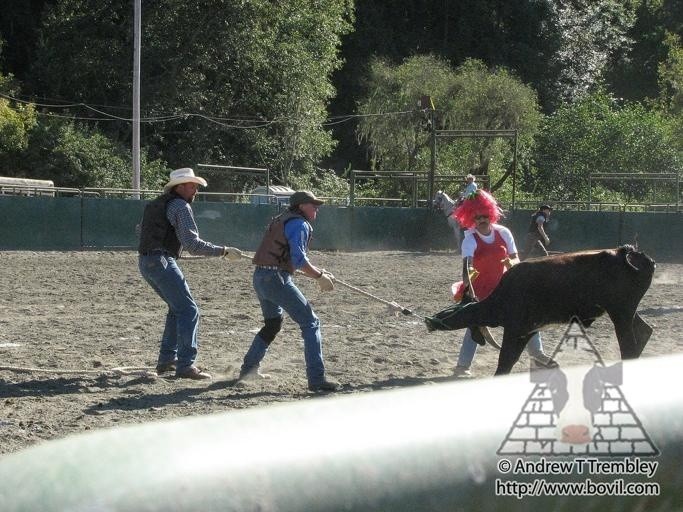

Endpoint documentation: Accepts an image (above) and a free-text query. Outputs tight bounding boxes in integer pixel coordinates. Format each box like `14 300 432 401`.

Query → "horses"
432 188 467 253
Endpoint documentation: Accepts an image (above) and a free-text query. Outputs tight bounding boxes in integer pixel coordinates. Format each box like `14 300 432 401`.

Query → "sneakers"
530 353 558 369
156 362 176 372
175 364 211 380
238 370 271 381
455 365 472 376
308 381 340 391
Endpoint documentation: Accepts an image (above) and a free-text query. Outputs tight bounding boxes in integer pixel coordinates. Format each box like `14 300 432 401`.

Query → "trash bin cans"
248 185 296 205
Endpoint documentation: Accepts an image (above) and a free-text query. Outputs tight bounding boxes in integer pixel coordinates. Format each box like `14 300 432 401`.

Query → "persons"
520 204 553 260
240 190 342 391
459 174 478 198
454 188 558 378
135 168 240 380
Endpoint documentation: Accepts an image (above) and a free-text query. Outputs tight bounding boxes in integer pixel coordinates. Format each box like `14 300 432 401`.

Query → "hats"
540 205 554 211
163 168 208 193
289 191 325 207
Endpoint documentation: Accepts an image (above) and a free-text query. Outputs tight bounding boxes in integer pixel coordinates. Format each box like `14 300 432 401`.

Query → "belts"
253 264 284 271
138 250 175 257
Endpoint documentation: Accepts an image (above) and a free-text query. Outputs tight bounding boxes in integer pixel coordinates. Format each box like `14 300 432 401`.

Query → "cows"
528 360 624 446
425 244 656 375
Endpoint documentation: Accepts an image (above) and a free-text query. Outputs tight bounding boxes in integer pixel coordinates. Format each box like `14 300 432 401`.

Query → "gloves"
220 246 242 262
320 269 336 285
314 272 334 293
